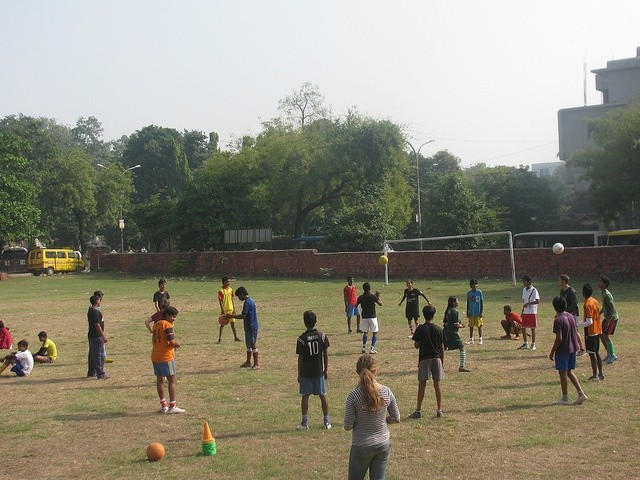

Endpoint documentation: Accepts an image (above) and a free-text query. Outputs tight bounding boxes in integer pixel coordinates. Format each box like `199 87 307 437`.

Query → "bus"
513 230 609 248
0 246 28 273
607 230 640 246
291 236 325 249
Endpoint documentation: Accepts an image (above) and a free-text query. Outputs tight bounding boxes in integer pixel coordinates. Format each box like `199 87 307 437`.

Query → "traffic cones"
201 421 216 456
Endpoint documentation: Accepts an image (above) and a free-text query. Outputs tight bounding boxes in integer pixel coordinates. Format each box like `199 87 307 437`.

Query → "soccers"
218 314 230 326
378 255 389 266
146 443 165 461
552 242 564 254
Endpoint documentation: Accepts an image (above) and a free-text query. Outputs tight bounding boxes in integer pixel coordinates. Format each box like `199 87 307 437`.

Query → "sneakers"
437 412 444 418
598 374 604 379
97 375 109 379
104 359 114 363
517 344 529 349
161 407 168 413
557 399 572 404
218 339 221 342
514 336 520 339
574 394 588 405
297 422 309 431
459 367 472 372
370 350 378 353
607 353 617 364
465 339 474 344
87 375 96 377
588 376 599 382
240 362 251 367
479 339 483 344
357 329 364 333
348 330 353 334
407 334 413 339
576 349 585 356
250 364 260 369
235 338 241 341
362 348 365 353
324 424 331 429
167 407 185 414
409 412 421 418
501 335 511 339
530 344 536 350
603 355 610 361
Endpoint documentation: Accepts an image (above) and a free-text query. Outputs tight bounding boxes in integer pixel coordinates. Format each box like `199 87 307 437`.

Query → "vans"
27 249 85 276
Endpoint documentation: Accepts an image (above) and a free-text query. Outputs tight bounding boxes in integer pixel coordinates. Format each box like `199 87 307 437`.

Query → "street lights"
96 164 141 253
404 139 436 251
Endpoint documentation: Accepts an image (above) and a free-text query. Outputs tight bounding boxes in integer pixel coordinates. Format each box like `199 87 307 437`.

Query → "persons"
295 310 332 431
516 275 540 350
442 295 472 373
465 279 484 345
86 296 111 380
354 282 383 354
0 321 13 349
576 283 605 382
145 298 170 333
153 279 170 311
222 286 260 370
558 273 586 357
599 277 618 364
548 296 588 406
217 276 242 343
151 306 187 414
398 279 432 338
343 275 364 334
342 354 401 480
88 291 114 363
501 305 532 340
32 331 58 364
408 305 445 420
0 340 34 377
